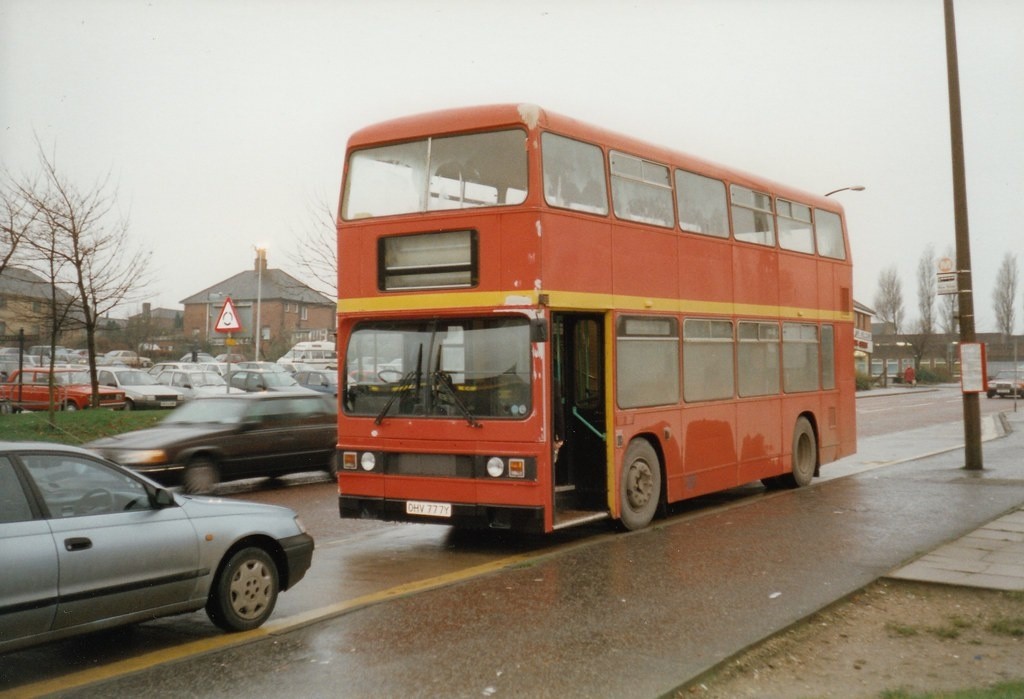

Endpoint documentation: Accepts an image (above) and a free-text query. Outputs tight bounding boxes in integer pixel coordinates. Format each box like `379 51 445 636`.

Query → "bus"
334 102 860 537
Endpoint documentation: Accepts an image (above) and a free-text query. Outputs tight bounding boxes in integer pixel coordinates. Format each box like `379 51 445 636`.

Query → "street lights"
824 186 866 197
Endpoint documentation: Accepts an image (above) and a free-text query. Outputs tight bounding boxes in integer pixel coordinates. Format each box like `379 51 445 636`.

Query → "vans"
275 340 338 372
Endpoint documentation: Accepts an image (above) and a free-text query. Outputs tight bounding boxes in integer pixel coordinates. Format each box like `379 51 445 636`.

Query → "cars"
0 368 125 410
0 345 152 373
155 369 246 400
80 391 338 498
350 370 386 385
152 353 285 375
350 357 402 373
86 366 185 411
220 369 301 394
986 369 1024 399
0 440 315 656
291 370 358 397
